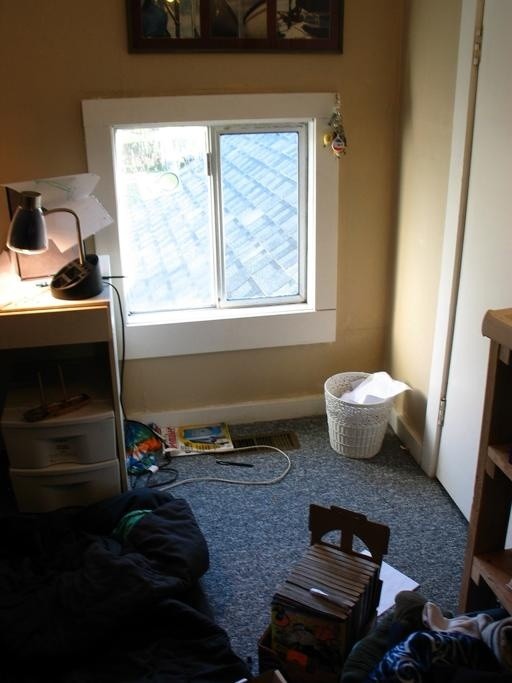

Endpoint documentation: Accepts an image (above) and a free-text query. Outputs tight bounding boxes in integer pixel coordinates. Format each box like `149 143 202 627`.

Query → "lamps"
5 191 102 299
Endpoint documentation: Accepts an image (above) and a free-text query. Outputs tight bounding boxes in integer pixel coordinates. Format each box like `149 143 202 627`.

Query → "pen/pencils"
215 458 255 471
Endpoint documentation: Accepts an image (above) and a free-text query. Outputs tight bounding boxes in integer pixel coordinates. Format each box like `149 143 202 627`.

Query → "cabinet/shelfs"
457 309 512 643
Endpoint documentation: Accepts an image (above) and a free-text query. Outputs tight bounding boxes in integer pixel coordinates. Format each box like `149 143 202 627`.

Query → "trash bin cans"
323 370 392 461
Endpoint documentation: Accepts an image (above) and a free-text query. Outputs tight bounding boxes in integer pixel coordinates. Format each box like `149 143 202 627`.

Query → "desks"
0 255 131 522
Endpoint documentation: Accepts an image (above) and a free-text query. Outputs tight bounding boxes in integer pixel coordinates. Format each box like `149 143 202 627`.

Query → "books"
270 541 381 662
160 421 234 456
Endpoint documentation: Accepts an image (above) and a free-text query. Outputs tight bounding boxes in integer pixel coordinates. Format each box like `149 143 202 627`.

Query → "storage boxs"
258 495 391 677
2 365 116 468
10 459 121 515
